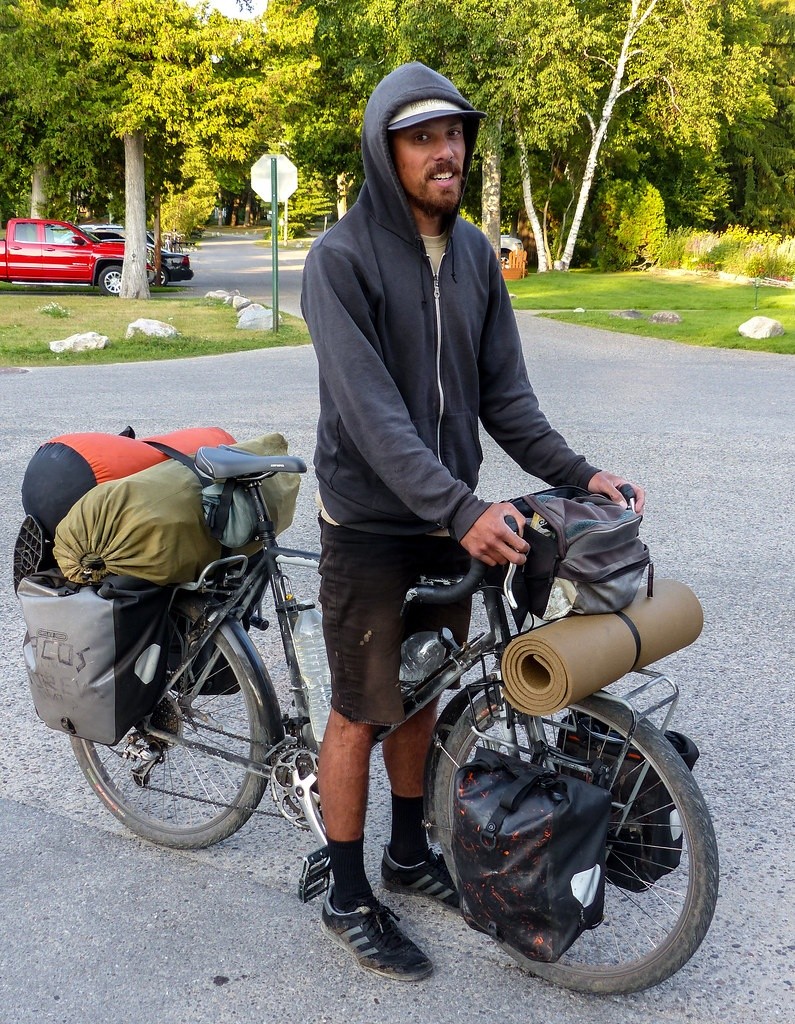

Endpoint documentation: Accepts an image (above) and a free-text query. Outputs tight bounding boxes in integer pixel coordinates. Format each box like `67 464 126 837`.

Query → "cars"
54 223 194 288
500 234 524 269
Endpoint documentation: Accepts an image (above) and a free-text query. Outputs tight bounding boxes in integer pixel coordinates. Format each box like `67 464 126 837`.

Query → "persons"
300 62 646 981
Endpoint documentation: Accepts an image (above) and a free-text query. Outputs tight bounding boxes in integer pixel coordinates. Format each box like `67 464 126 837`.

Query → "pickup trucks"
0 216 155 297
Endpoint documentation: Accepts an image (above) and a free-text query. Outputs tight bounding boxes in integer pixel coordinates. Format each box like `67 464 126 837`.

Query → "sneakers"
380 838 463 918
319 879 435 982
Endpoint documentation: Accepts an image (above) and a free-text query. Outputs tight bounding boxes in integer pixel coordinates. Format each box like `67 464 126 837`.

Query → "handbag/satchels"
168 616 251 697
555 710 701 893
450 744 613 964
17 565 172 747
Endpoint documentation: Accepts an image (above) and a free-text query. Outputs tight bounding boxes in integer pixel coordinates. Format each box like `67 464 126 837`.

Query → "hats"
384 99 491 133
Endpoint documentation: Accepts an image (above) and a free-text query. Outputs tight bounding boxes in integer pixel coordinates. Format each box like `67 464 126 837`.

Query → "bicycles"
17 440 724 1001
